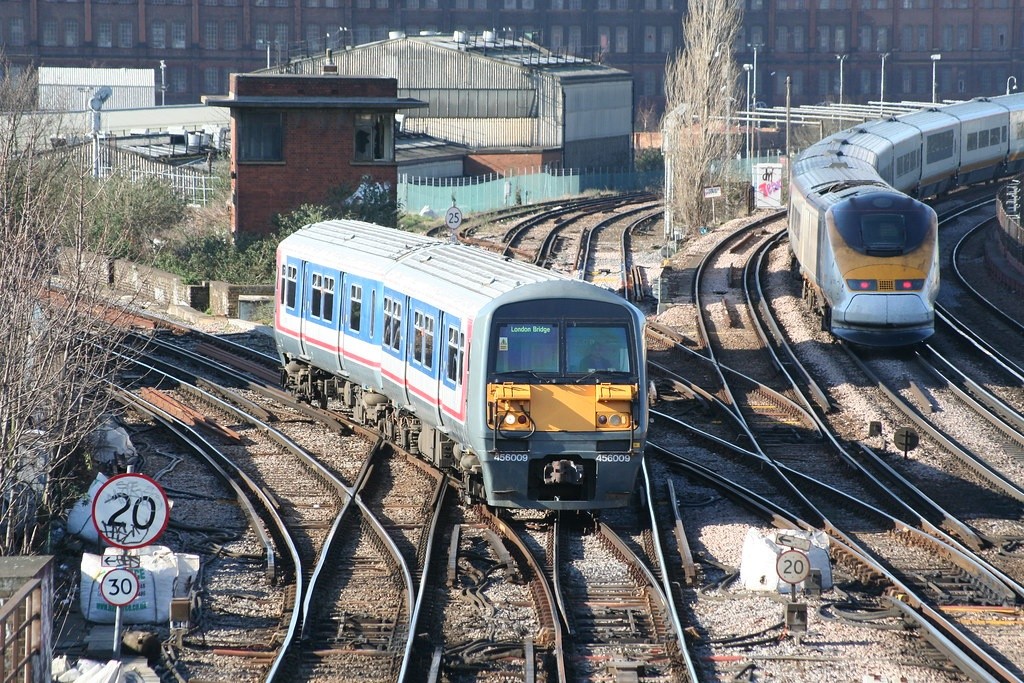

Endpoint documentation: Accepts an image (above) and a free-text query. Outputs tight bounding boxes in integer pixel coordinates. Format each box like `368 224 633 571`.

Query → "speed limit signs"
91 474 171 548
101 569 140 607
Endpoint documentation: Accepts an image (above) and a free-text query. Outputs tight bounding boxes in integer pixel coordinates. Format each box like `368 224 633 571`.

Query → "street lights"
712 41 1018 223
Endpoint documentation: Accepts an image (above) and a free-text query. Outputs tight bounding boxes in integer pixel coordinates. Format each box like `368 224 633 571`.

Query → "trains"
270 218 652 510
786 92 1023 350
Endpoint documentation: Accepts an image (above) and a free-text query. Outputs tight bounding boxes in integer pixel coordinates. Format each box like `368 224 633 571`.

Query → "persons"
580 341 612 372
